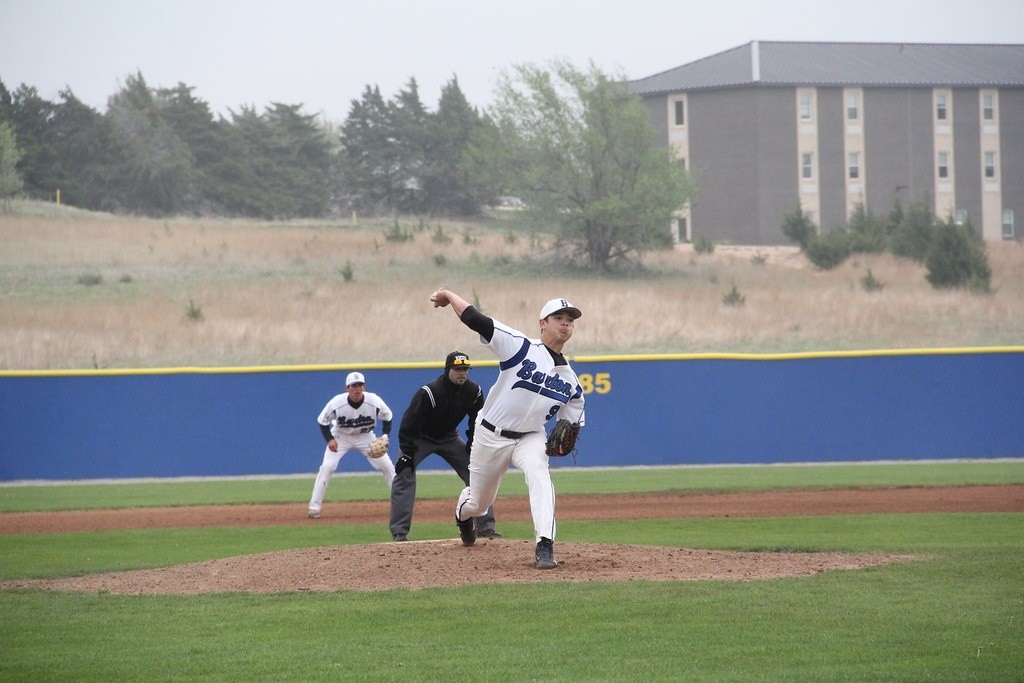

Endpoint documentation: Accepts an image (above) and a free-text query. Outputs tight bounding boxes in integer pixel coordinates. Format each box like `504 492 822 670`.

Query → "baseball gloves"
367 434 389 458
545 418 580 456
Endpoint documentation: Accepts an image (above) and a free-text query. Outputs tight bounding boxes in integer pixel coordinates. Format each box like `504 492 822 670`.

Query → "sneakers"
393 533 408 542
308 509 320 519
534 537 557 569
455 512 476 546
473 528 502 537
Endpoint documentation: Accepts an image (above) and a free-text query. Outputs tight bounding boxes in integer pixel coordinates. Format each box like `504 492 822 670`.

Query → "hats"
540 298 582 321
445 351 472 370
346 371 366 386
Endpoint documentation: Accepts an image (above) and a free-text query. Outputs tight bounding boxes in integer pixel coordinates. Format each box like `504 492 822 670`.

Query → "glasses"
446 360 470 368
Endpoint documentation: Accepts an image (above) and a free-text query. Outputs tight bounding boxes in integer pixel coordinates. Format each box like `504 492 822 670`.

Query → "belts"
480 419 535 439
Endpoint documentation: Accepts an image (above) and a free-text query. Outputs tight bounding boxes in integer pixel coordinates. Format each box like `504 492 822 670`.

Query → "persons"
430 286 586 570
389 352 503 542
308 372 397 518
